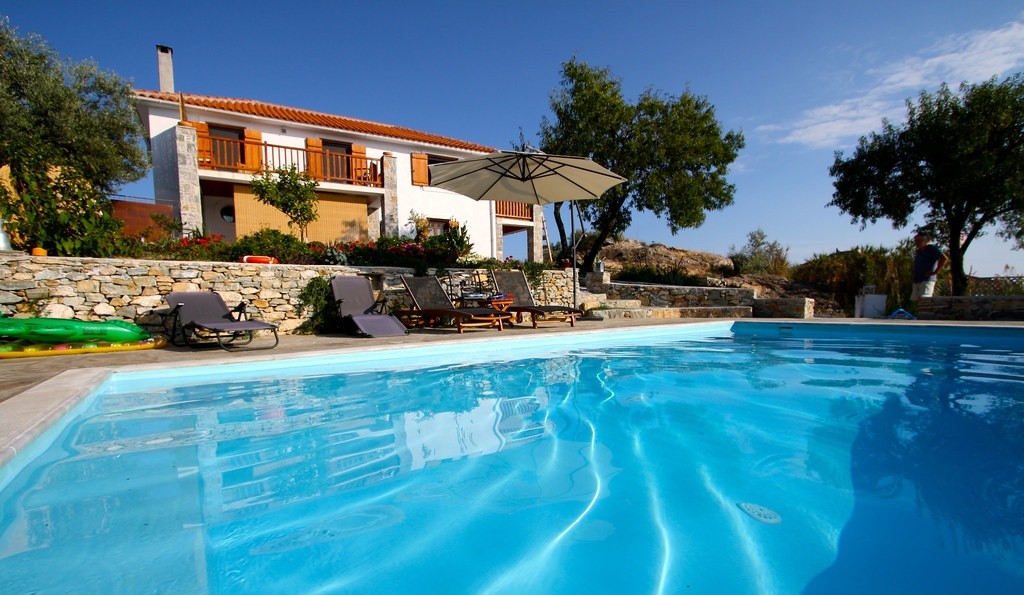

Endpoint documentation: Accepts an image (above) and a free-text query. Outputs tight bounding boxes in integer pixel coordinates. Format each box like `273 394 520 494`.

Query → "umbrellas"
428 148 627 262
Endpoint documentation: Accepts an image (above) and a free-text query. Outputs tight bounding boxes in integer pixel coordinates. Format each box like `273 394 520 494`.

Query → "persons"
910 232 948 313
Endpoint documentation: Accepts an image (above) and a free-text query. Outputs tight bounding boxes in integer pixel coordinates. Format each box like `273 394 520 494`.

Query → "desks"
454 298 514 328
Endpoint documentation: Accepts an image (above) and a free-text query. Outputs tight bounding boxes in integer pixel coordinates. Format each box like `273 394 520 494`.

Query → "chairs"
153 292 279 352
324 276 410 338
490 268 584 329
398 275 514 334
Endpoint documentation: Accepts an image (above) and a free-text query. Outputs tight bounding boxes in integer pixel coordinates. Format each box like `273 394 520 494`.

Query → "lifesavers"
240 253 279 264
